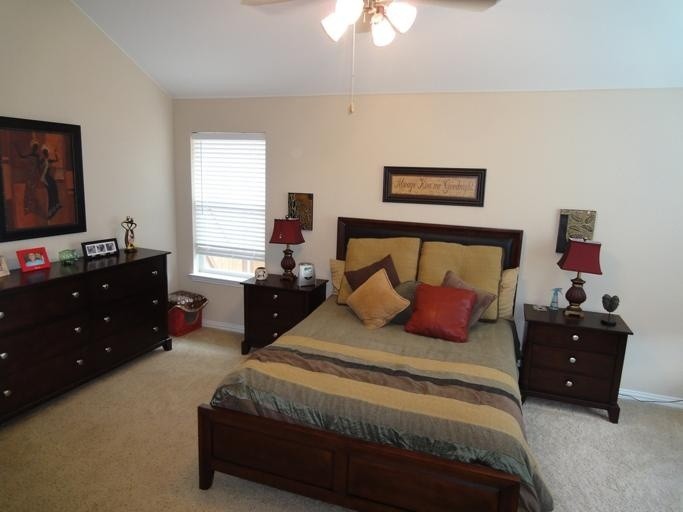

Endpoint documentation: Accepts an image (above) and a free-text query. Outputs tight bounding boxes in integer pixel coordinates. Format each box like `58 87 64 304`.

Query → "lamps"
269 219 305 282
322 0 418 114
557 237 602 317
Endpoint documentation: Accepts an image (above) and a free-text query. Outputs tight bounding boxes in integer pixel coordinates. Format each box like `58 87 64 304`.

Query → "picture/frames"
556 210 597 253
288 193 313 231
16 247 51 273
82 238 119 260
0 116 87 243
382 167 487 207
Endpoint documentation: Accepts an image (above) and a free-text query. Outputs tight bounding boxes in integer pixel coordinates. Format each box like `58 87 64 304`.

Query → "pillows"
417 241 504 321
497 268 518 318
405 284 477 343
337 237 420 306
346 269 411 330
441 271 497 332
330 258 344 293
343 254 400 293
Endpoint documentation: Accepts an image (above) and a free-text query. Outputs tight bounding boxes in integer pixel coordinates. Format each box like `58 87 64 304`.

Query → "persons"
26 253 43 266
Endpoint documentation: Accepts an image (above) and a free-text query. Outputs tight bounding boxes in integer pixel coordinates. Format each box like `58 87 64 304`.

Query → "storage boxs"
168 291 202 336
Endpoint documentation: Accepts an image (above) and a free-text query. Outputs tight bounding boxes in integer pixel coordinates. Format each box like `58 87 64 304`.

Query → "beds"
198 216 523 512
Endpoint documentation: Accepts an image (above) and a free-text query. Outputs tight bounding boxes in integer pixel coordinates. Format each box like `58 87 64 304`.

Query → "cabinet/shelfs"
0 247 172 422
238 273 329 356
519 304 633 424
